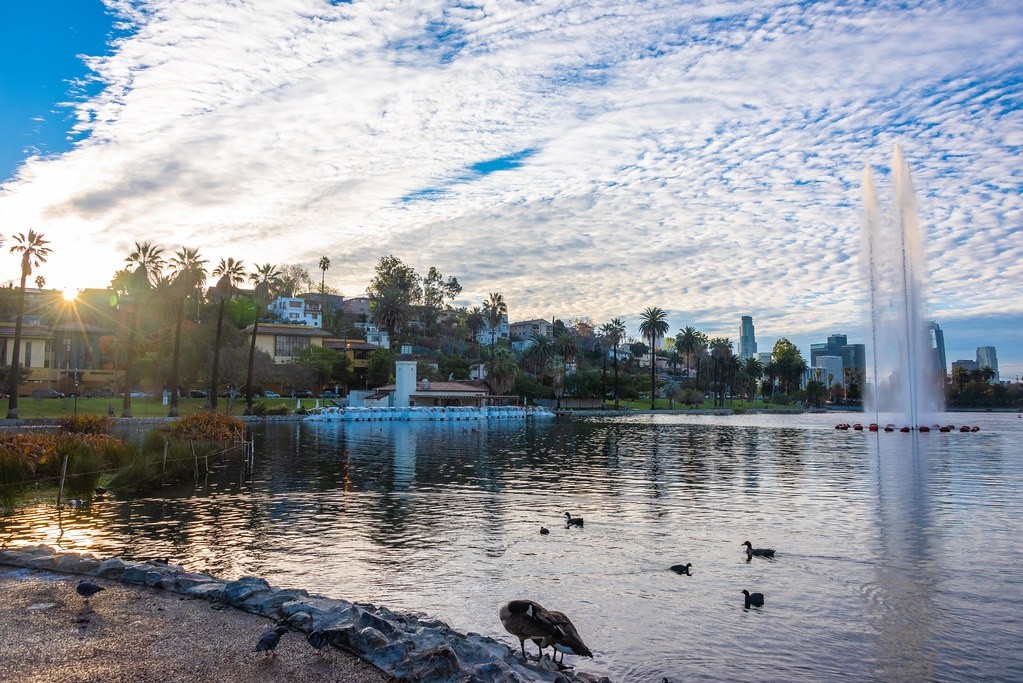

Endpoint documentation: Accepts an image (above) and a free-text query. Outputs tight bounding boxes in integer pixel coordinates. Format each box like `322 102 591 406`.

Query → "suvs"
32 390 65 400
86 388 112 399
290 390 313 398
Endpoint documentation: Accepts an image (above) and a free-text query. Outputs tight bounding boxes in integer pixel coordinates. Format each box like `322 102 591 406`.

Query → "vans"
189 390 207 398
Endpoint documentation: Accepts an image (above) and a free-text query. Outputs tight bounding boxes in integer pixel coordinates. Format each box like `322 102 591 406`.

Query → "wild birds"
461 426 476 433
251 624 348 659
670 562 694 576
564 512 584 529
741 541 777 558
77 579 105 602
500 599 593 668
540 527 549 534
94 488 107 497
740 589 765 610
108 404 116 417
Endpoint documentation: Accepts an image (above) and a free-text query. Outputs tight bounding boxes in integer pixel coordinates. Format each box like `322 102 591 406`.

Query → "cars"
0 391 10 399
263 391 280 399
220 390 241 398
318 391 340 398
117 390 144 399
